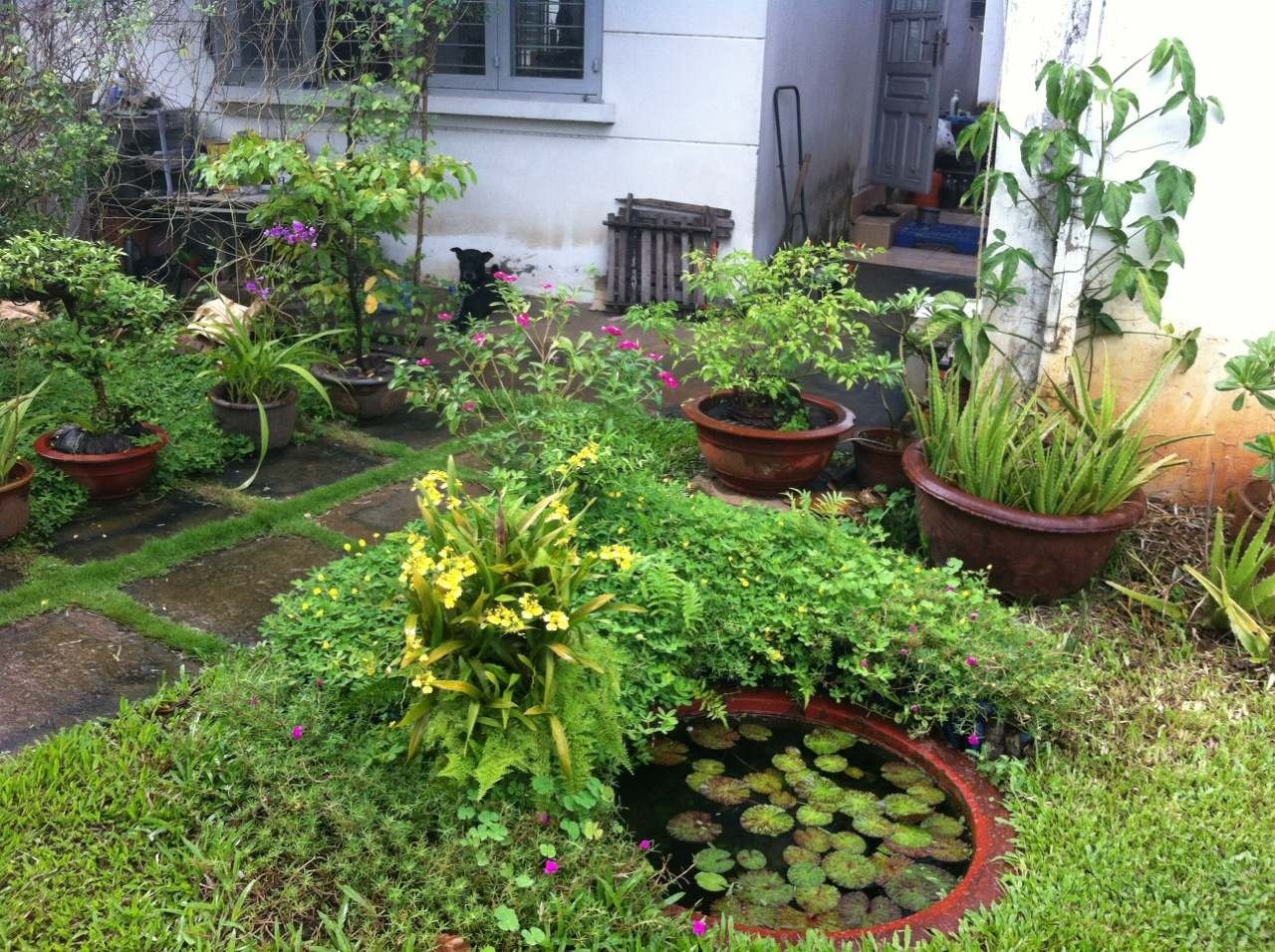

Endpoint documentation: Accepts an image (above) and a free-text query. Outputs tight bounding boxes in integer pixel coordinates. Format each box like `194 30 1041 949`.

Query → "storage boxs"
850 180 915 247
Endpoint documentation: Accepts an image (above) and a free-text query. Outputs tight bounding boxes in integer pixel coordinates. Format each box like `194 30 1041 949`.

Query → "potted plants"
625 237 881 500
345 43 458 341
902 339 1192 595
190 277 355 490
1215 327 1275 573
853 287 942 487
0 372 65 541
193 127 480 423
0 228 176 498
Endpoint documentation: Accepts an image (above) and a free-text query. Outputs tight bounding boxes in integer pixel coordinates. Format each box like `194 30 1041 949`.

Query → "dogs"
450 247 505 336
